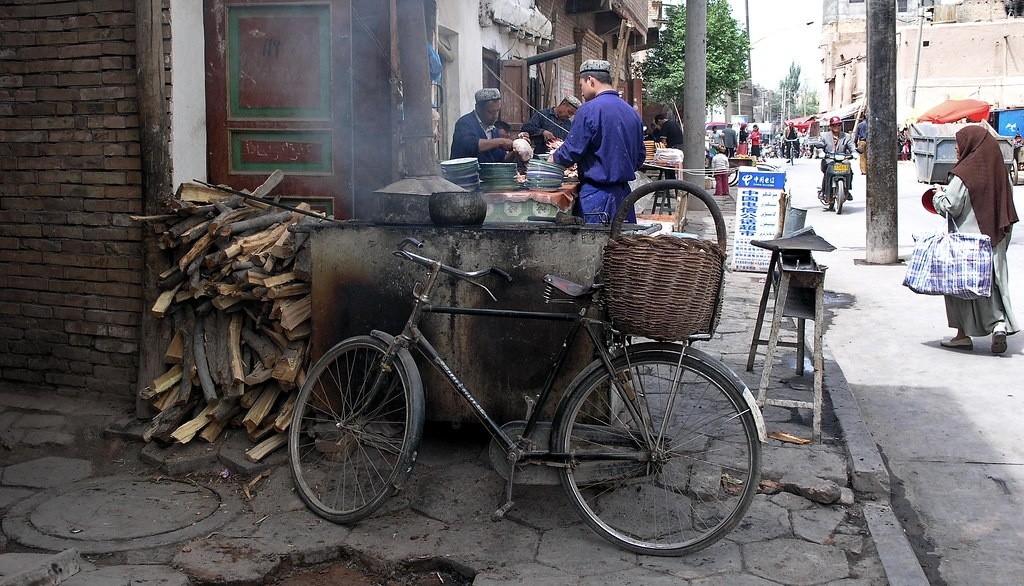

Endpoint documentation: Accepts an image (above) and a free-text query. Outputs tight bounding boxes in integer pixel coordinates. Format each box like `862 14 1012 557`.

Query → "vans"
705 122 725 136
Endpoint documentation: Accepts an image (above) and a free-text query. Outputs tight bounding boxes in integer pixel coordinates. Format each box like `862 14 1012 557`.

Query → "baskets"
601 179 727 338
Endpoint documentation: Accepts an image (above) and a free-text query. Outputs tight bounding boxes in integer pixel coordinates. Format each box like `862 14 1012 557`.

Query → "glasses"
830 123 841 127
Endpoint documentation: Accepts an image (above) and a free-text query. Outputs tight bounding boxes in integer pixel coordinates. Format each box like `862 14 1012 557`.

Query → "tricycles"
702 155 757 187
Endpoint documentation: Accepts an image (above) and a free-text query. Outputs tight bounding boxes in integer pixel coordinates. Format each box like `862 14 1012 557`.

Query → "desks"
641 166 678 215
485 182 580 221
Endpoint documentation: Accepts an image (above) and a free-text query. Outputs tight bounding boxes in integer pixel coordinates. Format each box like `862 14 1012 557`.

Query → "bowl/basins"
922 188 938 214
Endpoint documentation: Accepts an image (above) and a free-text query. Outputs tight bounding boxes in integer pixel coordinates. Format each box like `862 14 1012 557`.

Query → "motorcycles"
815 144 864 215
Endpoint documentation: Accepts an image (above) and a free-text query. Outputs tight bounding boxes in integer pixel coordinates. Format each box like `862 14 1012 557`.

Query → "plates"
523 159 566 191
440 157 481 192
643 141 655 159
479 163 518 192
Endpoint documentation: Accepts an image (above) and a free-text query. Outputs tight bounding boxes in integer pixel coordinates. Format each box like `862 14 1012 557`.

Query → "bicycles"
784 138 799 165
287 238 769 559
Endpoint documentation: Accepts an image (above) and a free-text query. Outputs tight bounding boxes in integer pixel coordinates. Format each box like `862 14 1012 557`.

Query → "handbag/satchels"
903 211 992 299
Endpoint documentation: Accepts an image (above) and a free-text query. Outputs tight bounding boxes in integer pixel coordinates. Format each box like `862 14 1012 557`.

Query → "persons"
709 122 807 163
710 146 731 196
642 112 684 179
932 125 1021 355
1013 134 1024 149
899 128 909 161
854 113 868 175
520 95 583 161
547 59 647 225
449 87 533 164
816 115 856 200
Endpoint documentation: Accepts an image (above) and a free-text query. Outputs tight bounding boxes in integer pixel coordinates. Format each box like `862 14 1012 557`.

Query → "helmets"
828 116 842 125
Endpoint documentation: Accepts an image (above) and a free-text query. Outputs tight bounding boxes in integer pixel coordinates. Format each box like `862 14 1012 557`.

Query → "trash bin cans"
911 118 1014 185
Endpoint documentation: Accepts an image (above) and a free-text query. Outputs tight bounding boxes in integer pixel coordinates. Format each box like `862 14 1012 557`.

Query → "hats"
475 88 501 102
580 59 611 74
565 96 582 108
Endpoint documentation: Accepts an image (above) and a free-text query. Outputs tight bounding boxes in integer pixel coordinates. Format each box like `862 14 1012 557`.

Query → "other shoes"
992 325 1008 353
846 192 852 200
786 160 791 163
940 336 973 351
